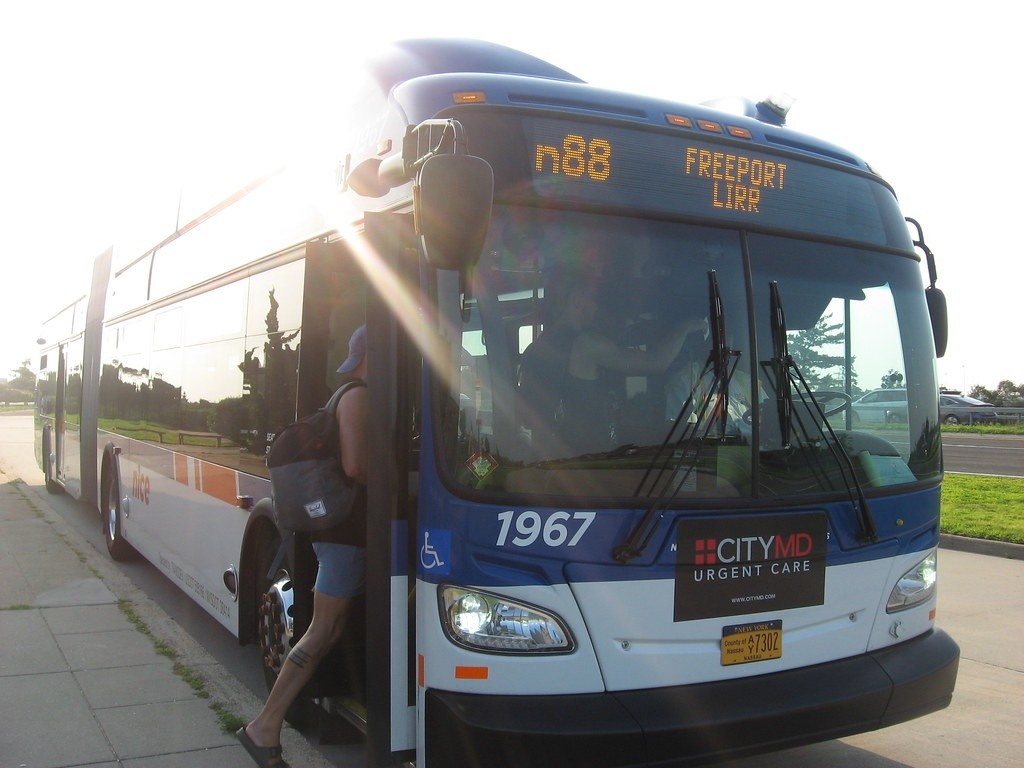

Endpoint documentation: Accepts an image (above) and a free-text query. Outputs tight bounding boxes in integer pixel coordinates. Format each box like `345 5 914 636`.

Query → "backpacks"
266 379 368 533
520 324 582 416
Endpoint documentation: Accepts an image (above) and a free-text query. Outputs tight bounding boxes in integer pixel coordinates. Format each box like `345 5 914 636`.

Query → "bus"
34 35 963 767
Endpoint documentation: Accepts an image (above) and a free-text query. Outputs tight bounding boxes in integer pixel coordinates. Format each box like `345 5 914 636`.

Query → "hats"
336 323 366 373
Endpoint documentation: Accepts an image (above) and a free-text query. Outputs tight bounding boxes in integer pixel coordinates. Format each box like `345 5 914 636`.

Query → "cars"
842 387 908 425
885 391 998 426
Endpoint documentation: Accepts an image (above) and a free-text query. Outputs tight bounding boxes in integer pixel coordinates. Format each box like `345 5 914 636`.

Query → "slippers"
236 727 292 768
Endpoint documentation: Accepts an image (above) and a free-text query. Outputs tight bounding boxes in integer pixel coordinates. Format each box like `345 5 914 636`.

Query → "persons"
516 261 709 467
238 323 400 768
